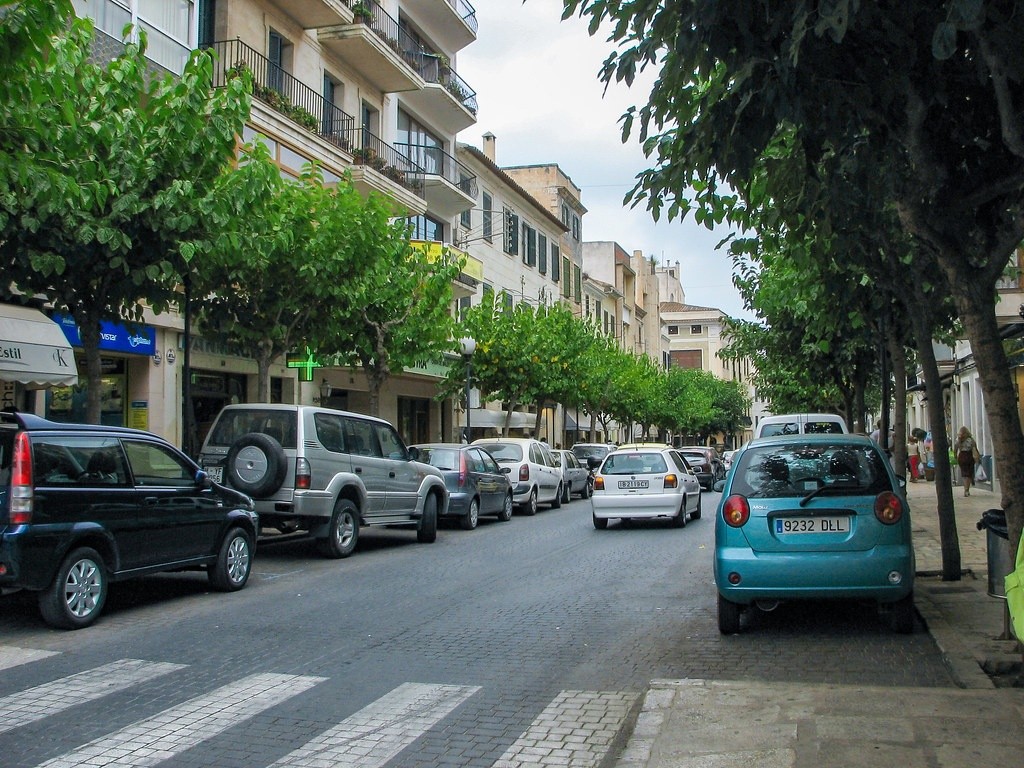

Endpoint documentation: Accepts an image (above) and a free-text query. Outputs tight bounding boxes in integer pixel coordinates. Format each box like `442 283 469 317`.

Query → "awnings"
0 303 78 387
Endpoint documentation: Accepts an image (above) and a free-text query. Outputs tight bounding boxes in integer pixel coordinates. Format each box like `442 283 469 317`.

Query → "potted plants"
353 147 387 169
347 0 378 24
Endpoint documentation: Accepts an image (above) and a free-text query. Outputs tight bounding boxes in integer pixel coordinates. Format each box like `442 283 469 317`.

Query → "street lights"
459 328 477 444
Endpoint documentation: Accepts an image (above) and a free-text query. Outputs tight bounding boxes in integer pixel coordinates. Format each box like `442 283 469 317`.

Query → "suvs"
194 403 451 559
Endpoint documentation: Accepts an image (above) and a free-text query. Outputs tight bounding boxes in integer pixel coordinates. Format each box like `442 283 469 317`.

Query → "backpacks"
1004 530 1024 644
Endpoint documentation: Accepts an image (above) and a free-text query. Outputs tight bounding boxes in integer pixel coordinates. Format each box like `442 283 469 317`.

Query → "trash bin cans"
982 509 1012 600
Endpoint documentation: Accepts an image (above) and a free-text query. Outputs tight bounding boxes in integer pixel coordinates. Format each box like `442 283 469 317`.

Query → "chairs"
625 459 644 472
349 434 365 455
829 450 860 485
88 451 117 476
650 463 663 472
757 456 792 492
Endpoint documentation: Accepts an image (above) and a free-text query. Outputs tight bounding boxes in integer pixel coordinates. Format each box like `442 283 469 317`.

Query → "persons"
870 420 895 448
954 426 979 497
529 431 535 439
907 428 933 483
540 437 546 442
523 433 529 438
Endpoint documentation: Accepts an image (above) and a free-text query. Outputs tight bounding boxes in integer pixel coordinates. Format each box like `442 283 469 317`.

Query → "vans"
753 413 850 439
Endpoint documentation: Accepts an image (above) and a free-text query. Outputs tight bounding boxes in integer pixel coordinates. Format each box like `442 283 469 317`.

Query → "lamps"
454 396 467 414
458 208 514 248
312 378 332 405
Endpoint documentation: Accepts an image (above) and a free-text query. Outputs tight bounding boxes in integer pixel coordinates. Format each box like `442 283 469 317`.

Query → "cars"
617 441 677 450
403 443 514 530
591 449 703 528
1 410 260 631
569 443 739 492
711 432 916 635
549 449 595 503
468 438 563 516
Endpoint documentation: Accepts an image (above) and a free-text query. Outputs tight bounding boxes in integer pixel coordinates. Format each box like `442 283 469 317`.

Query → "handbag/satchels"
974 464 988 482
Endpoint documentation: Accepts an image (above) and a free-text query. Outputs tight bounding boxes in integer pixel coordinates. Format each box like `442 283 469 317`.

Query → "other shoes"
912 478 917 483
909 478 913 482
964 491 970 496
